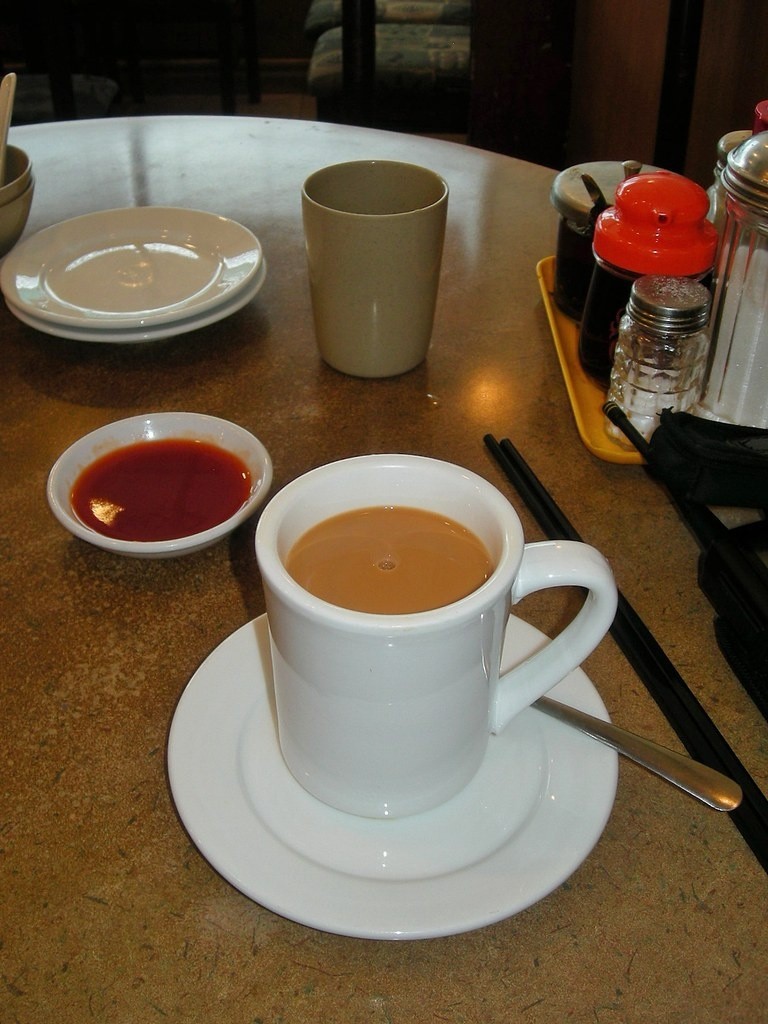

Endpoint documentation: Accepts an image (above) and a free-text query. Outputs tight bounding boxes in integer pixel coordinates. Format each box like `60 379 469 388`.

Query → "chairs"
303 0 561 168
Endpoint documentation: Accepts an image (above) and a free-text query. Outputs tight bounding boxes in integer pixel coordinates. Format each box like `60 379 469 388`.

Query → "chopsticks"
481 428 768 886
604 398 768 633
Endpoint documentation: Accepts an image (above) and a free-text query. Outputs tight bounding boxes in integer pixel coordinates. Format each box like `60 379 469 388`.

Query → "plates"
160 613 619 940
1 206 268 349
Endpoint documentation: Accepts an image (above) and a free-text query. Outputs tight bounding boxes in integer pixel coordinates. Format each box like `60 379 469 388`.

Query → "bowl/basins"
43 407 274 556
0 149 35 260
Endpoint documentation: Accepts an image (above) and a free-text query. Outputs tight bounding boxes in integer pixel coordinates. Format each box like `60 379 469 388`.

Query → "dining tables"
0 112 768 1019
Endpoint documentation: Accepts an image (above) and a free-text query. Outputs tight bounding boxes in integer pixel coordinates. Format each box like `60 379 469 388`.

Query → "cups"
255 452 617 817
557 106 767 442
301 157 444 381
